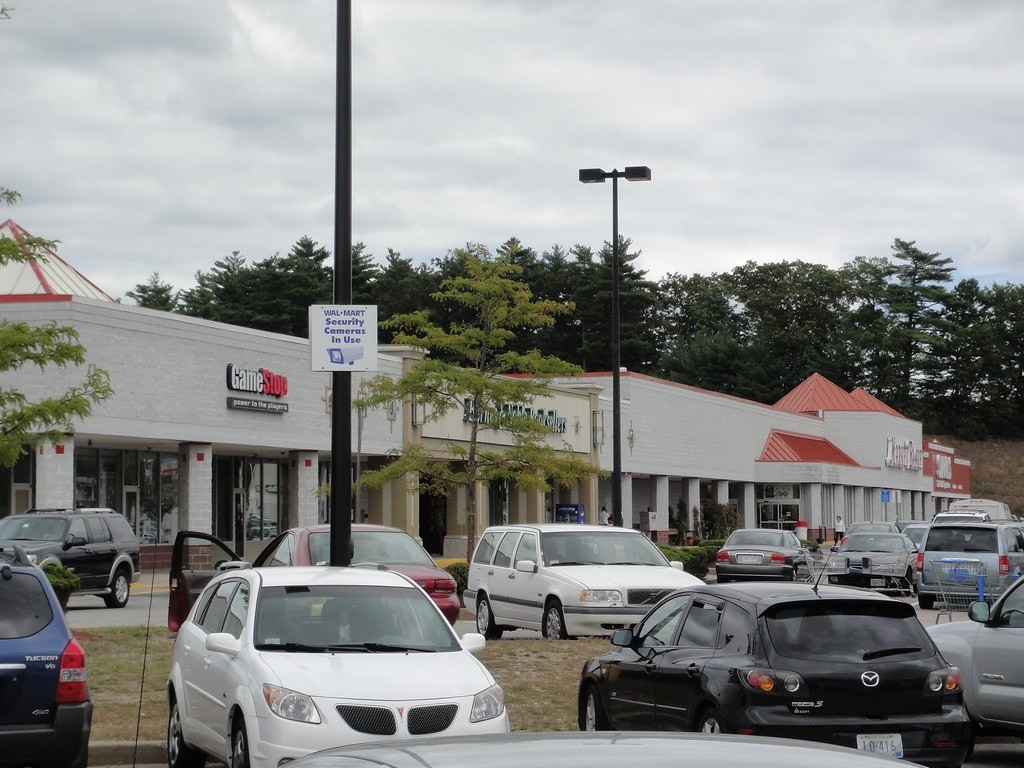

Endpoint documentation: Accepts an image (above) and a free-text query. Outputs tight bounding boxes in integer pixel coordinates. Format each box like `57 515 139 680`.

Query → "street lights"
582 165 654 524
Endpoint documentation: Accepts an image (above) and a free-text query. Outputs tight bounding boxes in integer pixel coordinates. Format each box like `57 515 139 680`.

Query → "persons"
835 515 845 540
599 506 610 525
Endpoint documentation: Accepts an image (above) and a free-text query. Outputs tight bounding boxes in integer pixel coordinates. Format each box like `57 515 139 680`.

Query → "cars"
576 579 976 768
828 533 916 593
0 548 93 768
166 561 510 768
169 521 460 638
926 575 1023 734
244 515 278 537
160 508 179 543
842 519 930 550
715 529 814 582
141 512 158 543
273 731 929 767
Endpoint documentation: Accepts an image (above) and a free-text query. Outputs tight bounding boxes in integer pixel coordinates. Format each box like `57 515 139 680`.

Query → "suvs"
916 519 1023 609
465 523 714 642
0 505 141 609
932 511 994 547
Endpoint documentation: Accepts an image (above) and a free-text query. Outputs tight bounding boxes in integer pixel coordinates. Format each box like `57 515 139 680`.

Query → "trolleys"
863 547 918 598
927 556 1020 627
797 547 837 584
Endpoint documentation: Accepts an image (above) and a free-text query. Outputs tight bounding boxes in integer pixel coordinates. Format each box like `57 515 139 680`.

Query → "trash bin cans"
795 521 808 540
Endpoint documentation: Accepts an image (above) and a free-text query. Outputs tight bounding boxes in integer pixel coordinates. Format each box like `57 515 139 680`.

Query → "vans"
948 499 1013 521
